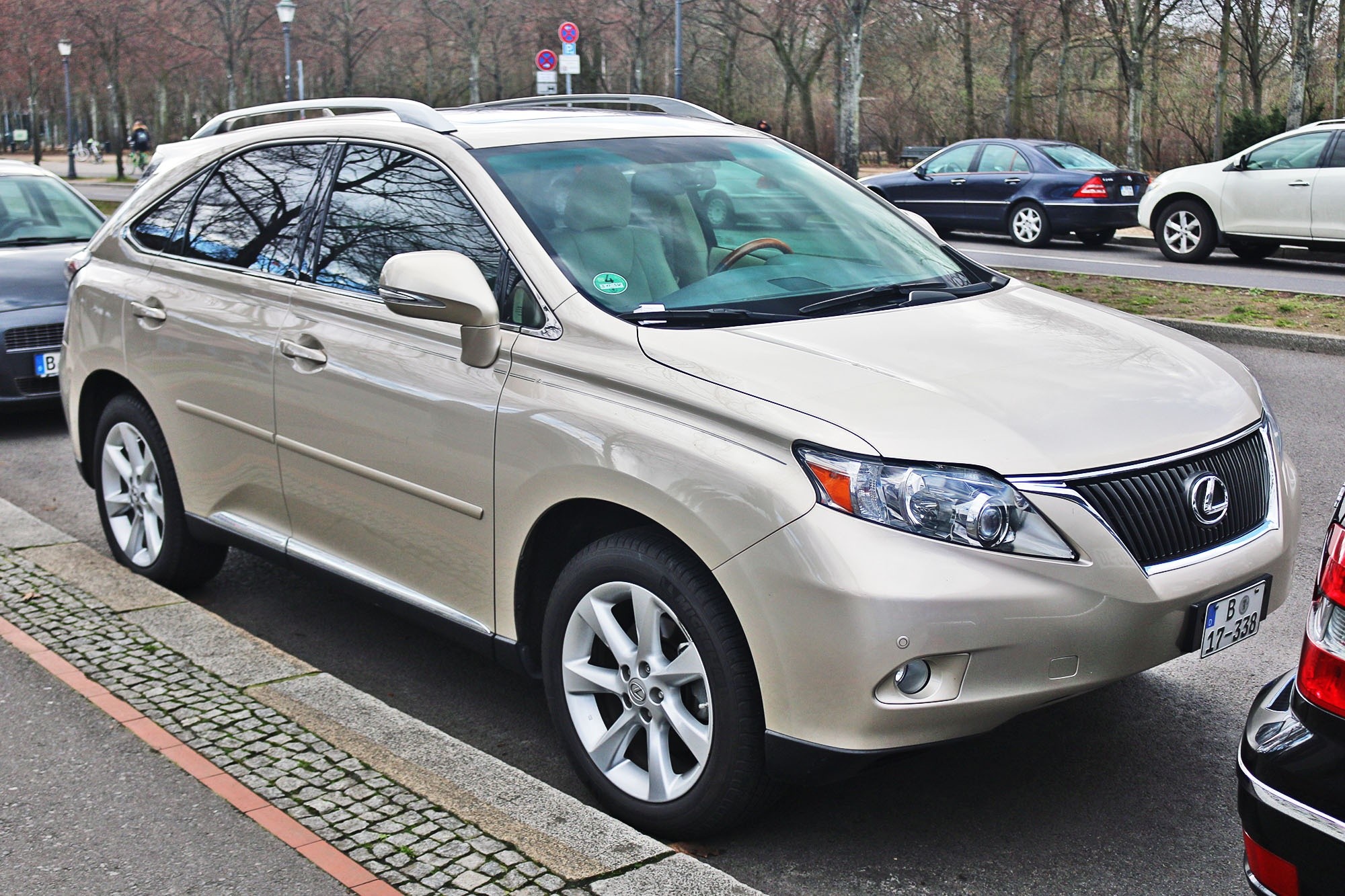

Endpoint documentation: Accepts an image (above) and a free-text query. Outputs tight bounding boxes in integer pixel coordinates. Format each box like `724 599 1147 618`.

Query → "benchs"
900 146 945 168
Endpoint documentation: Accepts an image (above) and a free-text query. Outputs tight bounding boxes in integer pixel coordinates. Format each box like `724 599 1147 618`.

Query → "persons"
124 117 151 162
756 120 772 132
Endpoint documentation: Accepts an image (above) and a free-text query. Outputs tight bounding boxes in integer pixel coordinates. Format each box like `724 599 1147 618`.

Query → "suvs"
58 88 1300 842
1138 117 1344 268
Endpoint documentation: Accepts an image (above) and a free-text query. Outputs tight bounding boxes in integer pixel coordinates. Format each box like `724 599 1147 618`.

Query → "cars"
0 160 109 410
854 138 1151 248
1235 481 1345 896
544 147 828 232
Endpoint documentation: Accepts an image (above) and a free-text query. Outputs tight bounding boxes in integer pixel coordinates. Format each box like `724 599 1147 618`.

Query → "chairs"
977 154 1000 172
546 162 680 313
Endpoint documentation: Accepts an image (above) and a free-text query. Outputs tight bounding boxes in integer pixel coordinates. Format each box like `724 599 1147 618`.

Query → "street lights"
58 29 82 180
274 0 300 121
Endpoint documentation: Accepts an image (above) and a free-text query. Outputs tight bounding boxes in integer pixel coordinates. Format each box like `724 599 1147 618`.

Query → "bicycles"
124 140 150 175
72 137 109 164
